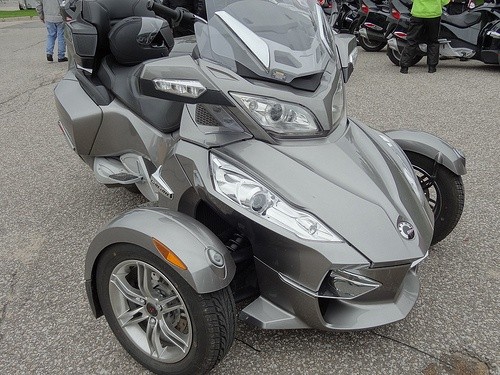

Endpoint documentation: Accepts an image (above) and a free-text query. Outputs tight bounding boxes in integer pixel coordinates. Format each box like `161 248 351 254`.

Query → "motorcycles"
356 1 393 51
387 1 499 67
333 1 360 30
49 1 466 375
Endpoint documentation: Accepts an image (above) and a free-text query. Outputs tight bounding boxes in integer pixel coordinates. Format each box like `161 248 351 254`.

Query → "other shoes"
46 53 53 61
399 66 408 74
58 57 68 62
427 65 436 73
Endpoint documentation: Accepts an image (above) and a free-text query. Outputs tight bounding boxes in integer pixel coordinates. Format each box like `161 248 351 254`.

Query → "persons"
35 0 70 62
400 0 451 74
